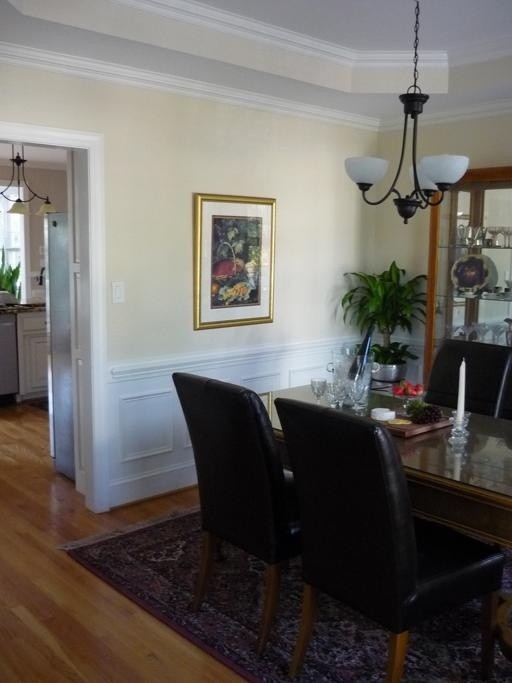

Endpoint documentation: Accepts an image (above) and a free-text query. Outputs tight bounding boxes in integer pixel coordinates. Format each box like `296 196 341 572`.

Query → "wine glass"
459 321 508 345
311 378 327 405
455 226 512 249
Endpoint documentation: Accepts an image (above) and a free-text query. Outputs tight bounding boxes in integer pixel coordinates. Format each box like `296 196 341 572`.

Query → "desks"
243 382 509 558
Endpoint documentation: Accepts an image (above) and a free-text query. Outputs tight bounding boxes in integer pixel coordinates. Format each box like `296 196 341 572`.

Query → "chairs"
423 338 509 420
172 371 297 659
272 395 509 682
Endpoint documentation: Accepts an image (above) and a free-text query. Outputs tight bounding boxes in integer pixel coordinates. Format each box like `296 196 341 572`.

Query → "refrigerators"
43 210 75 485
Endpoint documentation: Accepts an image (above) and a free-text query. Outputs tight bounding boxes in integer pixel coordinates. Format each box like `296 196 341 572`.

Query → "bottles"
349 318 377 385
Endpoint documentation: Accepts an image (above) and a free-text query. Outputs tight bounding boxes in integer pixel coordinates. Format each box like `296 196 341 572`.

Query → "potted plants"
340 260 429 395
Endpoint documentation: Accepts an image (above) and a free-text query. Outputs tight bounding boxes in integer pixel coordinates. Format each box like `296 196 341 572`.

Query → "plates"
452 255 491 294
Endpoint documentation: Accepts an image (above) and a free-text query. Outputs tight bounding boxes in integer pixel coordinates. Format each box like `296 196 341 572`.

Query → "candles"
456 357 466 425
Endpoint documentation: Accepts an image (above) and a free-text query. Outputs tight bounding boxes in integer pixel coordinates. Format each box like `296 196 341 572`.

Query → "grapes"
413 405 441 424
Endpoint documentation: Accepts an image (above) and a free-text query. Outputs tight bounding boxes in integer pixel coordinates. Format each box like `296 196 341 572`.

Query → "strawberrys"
393 380 424 397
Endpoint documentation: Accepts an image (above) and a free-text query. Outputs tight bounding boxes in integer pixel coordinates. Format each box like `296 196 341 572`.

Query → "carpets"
56 502 511 683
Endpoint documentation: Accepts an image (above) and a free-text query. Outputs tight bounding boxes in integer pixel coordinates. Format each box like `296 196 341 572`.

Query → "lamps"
0 144 56 218
343 1 470 226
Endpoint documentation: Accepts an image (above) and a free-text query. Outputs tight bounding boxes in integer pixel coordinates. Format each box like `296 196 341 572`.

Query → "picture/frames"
191 192 276 331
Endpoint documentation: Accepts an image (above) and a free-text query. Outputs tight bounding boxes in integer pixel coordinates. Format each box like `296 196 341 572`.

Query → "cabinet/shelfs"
432 180 512 363
15 312 48 403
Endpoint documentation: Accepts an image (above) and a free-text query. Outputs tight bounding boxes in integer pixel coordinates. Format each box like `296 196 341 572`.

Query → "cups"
327 383 343 409
